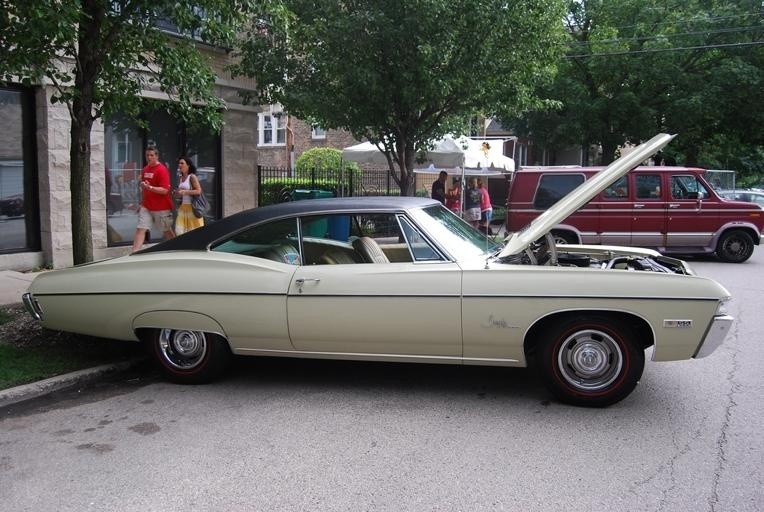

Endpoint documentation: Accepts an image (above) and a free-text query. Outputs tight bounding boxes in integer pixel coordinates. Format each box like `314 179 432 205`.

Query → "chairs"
266 237 388 265
684 191 704 198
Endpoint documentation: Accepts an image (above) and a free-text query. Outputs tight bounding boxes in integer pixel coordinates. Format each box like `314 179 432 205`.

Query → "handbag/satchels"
191 192 210 218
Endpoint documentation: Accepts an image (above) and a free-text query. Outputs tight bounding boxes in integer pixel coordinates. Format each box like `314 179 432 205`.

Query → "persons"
478 178 493 227
171 155 205 237
464 175 483 230
132 146 176 257
447 179 461 216
431 169 460 210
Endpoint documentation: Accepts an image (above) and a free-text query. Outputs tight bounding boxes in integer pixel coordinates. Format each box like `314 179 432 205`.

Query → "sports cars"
21 133 735 408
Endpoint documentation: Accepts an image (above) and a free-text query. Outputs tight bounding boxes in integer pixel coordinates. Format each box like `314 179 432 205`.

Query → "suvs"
505 164 764 264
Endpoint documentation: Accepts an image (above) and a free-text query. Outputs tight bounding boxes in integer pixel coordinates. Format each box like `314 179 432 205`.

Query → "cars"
0 193 123 218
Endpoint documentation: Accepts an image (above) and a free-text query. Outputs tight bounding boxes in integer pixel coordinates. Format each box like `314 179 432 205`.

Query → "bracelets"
148 185 153 190
